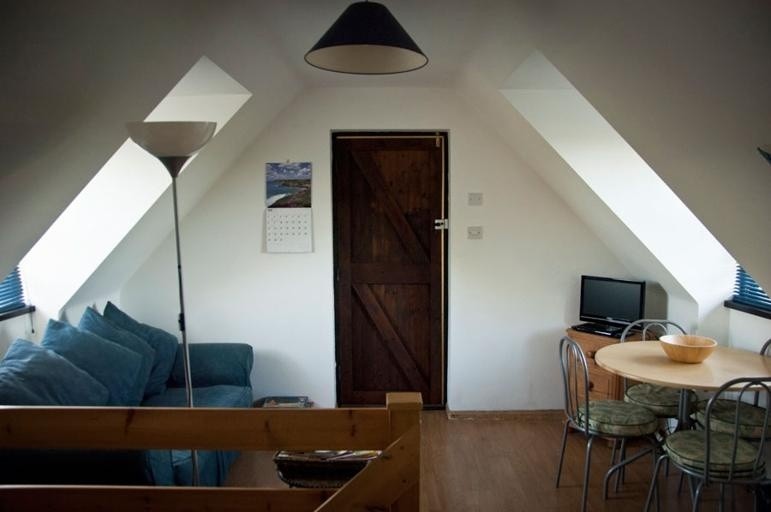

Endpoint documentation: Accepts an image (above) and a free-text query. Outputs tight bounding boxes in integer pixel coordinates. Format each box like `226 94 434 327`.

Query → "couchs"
0 342 253 487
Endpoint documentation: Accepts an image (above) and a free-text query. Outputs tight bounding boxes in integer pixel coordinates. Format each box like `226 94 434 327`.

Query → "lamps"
126 121 218 487
302 1 429 75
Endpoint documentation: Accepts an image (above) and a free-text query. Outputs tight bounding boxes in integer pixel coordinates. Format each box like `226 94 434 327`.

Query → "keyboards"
572 322 636 338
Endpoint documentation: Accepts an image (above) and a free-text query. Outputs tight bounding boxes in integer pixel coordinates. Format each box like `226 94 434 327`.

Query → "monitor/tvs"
580 275 646 330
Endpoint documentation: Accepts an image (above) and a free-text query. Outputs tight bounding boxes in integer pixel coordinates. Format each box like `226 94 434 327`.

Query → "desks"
595 340 771 512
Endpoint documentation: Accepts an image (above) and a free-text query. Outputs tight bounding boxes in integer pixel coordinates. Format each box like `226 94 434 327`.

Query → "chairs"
643 376 771 511
677 335 771 496
553 334 661 511
620 318 689 481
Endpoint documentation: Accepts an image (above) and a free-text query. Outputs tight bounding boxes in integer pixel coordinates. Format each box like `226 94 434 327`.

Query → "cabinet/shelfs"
566 329 654 451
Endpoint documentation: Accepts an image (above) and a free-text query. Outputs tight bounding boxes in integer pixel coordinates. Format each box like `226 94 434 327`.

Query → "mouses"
611 330 622 339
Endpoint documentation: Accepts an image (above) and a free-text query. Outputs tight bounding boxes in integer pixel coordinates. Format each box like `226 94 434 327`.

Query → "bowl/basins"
659 334 718 365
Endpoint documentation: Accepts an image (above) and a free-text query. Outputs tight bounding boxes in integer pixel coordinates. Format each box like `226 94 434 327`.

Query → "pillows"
41 320 144 407
79 308 157 407
103 301 179 395
2 338 110 406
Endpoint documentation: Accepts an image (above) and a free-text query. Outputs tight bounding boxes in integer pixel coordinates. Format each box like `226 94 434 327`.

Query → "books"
275 449 379 462
263 396 307 409
